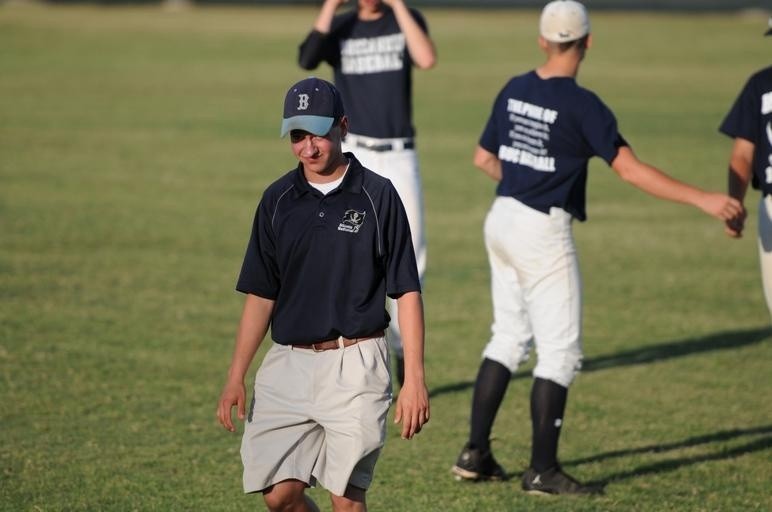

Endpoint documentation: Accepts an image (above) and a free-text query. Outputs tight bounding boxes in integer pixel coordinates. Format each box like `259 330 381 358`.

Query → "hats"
539 0 591 47
278 73 345 140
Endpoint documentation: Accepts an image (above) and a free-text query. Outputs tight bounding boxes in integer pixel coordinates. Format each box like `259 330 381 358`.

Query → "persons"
298 0 437 389
718 16 772 316
218 75 430 512
452 0 747 497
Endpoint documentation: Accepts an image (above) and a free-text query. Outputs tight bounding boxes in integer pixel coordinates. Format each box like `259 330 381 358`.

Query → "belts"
279 329 384 351
356 141 414 155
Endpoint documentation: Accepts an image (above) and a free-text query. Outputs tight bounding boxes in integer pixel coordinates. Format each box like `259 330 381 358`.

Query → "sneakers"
451 439 509 483
519 463 594 498
